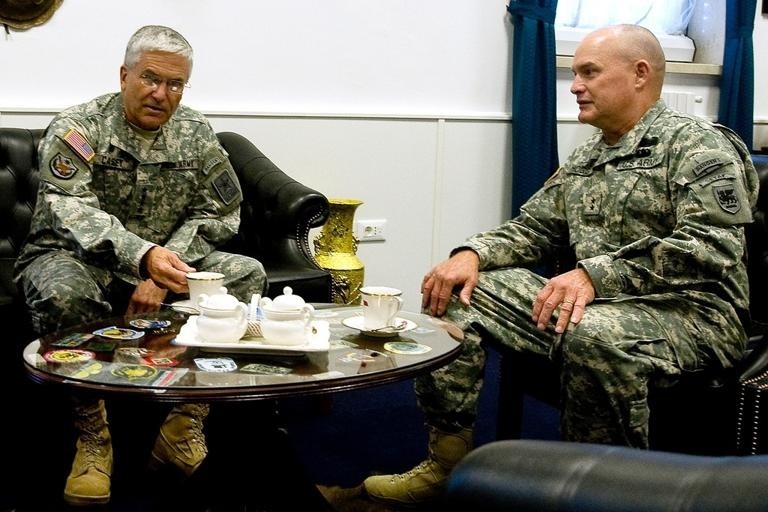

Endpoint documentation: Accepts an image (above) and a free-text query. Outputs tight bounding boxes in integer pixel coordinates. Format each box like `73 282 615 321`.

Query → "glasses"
129 69 191 94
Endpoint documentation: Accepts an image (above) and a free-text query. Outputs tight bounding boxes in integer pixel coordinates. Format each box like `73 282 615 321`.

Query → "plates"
169 300 199 314
342 315 416 337
171 315 331 356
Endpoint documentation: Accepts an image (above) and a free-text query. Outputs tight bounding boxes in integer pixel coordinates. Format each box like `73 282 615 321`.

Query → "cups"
360 286 403 330
185 273 224 307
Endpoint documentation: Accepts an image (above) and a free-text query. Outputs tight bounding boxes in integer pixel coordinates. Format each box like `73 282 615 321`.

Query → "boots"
64 401 111 506
152 400 209 477
362 422 473 510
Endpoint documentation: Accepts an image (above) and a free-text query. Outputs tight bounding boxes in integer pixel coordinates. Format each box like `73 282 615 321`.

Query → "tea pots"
198 287 249 343
259 287 315 345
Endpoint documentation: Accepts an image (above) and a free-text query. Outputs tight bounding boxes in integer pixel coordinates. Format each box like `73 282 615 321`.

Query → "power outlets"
357 219 387 243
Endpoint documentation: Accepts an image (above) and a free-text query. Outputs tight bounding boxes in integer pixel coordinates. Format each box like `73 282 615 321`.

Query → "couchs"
442 438 767 511
496 155 768 454
0 128 331 303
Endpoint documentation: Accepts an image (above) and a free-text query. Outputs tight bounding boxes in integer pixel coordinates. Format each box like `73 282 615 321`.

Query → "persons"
363 23 760 506
11 25 269 505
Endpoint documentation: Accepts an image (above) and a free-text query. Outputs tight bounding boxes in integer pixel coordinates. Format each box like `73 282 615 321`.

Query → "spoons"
370 324 405 332
160 302 201 312
